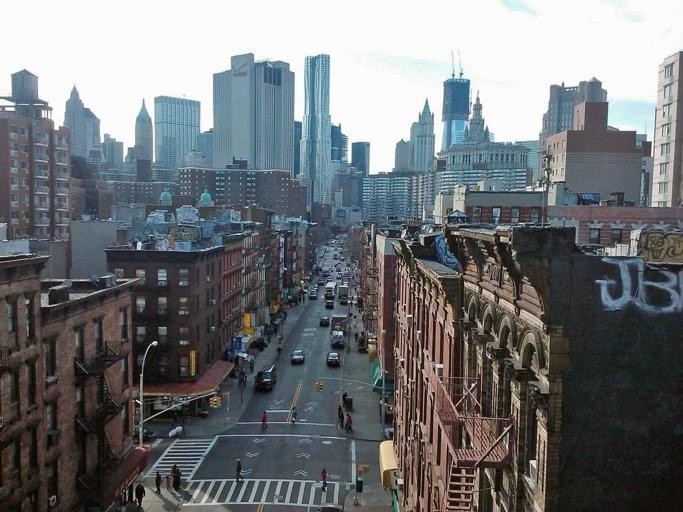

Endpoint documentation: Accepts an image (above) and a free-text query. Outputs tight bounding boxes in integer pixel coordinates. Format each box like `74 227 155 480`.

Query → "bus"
322 281 337 300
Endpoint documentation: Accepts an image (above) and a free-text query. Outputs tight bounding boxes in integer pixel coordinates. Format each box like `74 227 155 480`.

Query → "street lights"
137 340 218 480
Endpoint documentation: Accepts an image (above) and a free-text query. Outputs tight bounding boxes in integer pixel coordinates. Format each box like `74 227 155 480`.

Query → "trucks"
338 285 349 299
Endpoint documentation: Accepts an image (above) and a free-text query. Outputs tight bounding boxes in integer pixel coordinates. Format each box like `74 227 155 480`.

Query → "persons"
347 275 361 342
234 355 255 389
277 347 282 360
135 483 145 510
261 409 267 432
338 406 354 434
267 298 288 344
342 391 349 409
165 464 182 491
235 459 244 482
291 406 297 421
155 471 161 494
321 468 327 492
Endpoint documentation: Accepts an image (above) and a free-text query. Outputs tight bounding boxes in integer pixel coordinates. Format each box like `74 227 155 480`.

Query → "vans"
252 365 277 393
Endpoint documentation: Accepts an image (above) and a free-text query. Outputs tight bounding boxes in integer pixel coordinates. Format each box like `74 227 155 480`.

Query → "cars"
327 352 341 368
319 316 329 327
310 266 334 300
291 350 305 364
